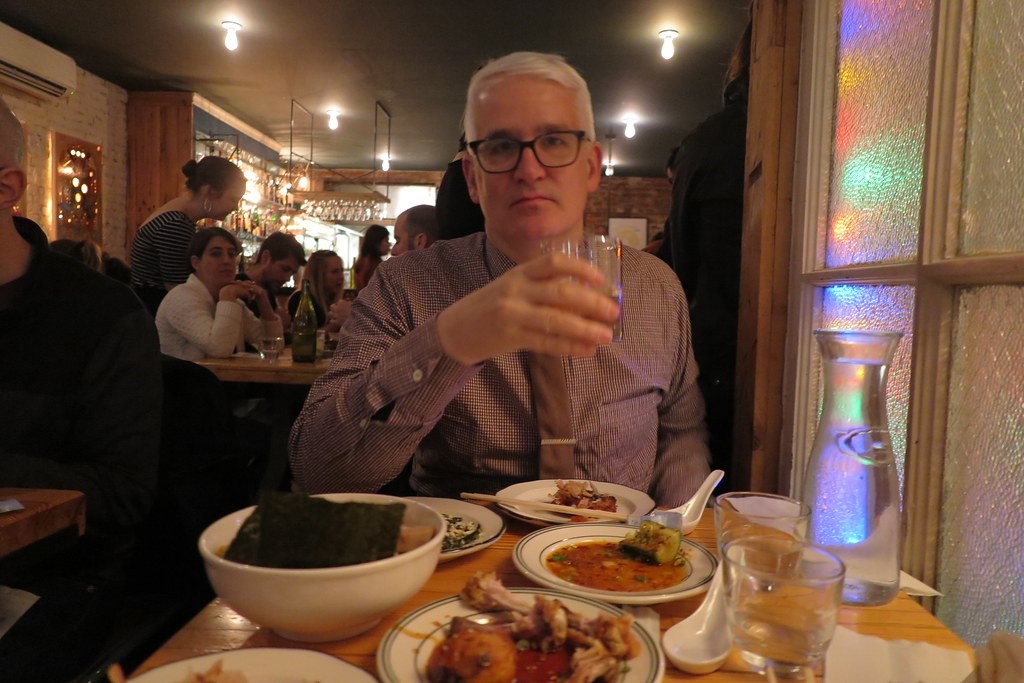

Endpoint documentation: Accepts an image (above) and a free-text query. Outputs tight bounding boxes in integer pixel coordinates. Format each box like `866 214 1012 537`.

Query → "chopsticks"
460 492 628 520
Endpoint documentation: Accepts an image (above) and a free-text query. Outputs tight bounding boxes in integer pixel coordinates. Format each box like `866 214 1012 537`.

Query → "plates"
128 649 378 683
496 479 656 526
511 522 718 604
379 587 667 683
398 497 504 559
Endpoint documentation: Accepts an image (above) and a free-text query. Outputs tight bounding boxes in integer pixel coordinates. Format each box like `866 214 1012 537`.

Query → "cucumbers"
620 519 682 563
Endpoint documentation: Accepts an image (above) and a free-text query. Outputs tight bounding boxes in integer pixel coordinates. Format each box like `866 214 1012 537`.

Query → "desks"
0 486 86 558
192 334 333 383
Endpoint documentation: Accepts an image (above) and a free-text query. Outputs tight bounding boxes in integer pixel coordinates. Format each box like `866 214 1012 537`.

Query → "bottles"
350 257 356 289
264 175 281 204
292 277 317 363
224 210 279 236
801 330 907 608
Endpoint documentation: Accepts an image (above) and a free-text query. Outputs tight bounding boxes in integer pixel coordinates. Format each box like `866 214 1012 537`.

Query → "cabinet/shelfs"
195 129 306 276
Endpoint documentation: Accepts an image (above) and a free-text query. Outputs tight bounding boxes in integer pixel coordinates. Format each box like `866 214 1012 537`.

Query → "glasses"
465 130 594 175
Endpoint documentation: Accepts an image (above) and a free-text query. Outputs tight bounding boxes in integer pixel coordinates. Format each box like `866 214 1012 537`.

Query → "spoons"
662 560 730 676
667 460 725 534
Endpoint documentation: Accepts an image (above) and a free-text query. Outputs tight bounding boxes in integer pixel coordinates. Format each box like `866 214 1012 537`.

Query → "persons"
154 227 285 362
651 0 752 496
435 132 487 240
329 205 436 326
235 231 344 353
287 51 713 507
130 155 246 313
354 225 392 292
0 98 162 683
49 239 131 286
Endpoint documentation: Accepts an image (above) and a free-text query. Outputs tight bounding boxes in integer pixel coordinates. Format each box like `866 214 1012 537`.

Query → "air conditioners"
0 21 77 101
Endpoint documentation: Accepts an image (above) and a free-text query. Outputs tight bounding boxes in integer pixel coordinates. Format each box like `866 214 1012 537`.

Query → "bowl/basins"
198 494 447 642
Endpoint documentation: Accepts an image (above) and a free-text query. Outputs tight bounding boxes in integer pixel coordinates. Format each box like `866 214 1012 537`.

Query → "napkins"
824 625 974 683
841 555 945 597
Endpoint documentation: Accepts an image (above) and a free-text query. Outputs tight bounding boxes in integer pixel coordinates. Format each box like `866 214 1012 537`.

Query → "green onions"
553 523 651 581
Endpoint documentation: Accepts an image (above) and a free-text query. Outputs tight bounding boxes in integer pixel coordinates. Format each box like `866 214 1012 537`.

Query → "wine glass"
258 313 284 368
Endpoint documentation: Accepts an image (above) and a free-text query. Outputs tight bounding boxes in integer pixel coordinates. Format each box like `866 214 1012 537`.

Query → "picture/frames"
607 216 649 250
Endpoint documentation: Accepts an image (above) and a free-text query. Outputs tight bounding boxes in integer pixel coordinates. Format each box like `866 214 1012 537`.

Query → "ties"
525 346 579 479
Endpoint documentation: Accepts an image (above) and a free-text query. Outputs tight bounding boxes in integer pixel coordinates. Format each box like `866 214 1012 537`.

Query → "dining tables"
128 501 981 683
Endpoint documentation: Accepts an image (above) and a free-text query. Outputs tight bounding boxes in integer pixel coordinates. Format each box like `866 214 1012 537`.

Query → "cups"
317 329 325 361
542 235 625 343
714 492 846 677
